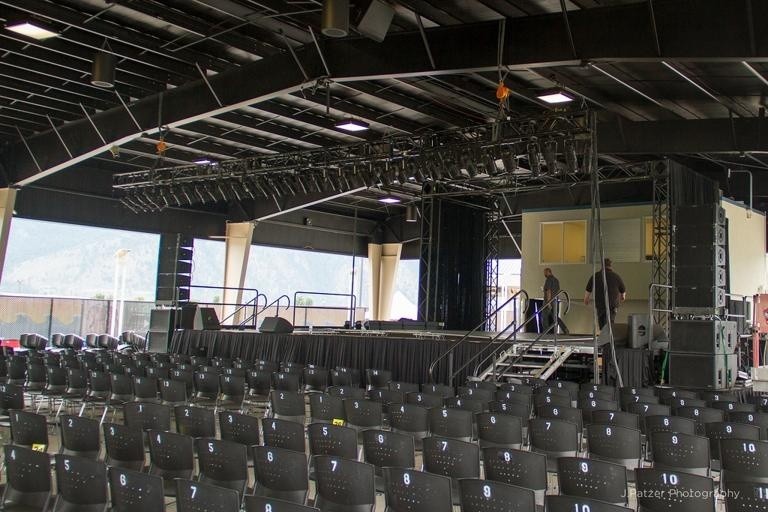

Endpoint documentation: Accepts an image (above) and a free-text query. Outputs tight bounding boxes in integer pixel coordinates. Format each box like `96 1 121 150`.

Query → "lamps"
322 0 348 38
402 203 418 223
533 87 576 107
111 107 595 215
90 38 117 90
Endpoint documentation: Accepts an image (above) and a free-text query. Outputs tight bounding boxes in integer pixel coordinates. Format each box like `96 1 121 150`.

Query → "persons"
584 258 626 331
542 268 560 334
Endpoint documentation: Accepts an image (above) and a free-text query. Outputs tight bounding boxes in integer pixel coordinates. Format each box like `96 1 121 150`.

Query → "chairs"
1 332 768 511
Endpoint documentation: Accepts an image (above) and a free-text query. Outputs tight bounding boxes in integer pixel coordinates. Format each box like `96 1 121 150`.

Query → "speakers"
258 316 295 334
148 212 197 358
194 307 220 330
668 175 738 392
